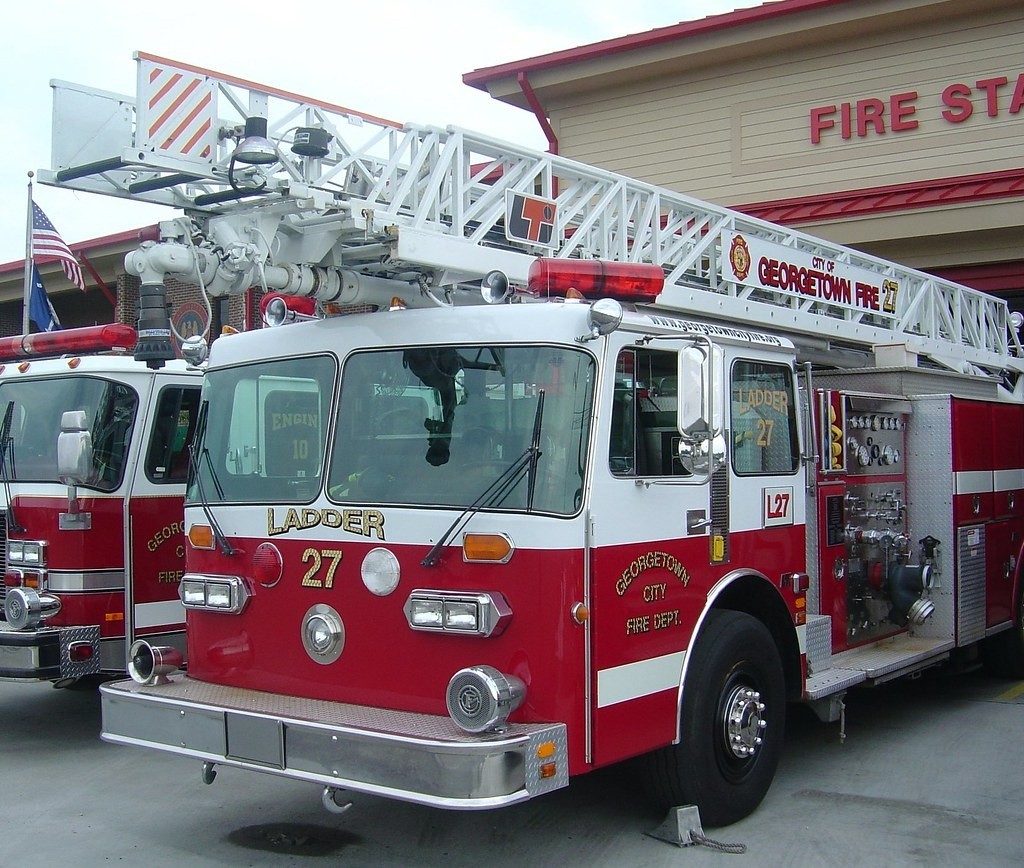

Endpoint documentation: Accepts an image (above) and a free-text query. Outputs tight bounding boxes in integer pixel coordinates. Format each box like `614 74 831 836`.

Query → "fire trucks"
1 323 646 690
35 51 1024 828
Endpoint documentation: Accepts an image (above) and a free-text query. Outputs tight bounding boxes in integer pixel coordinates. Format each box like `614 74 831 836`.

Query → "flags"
29 198 85 291
27 263 60 335
